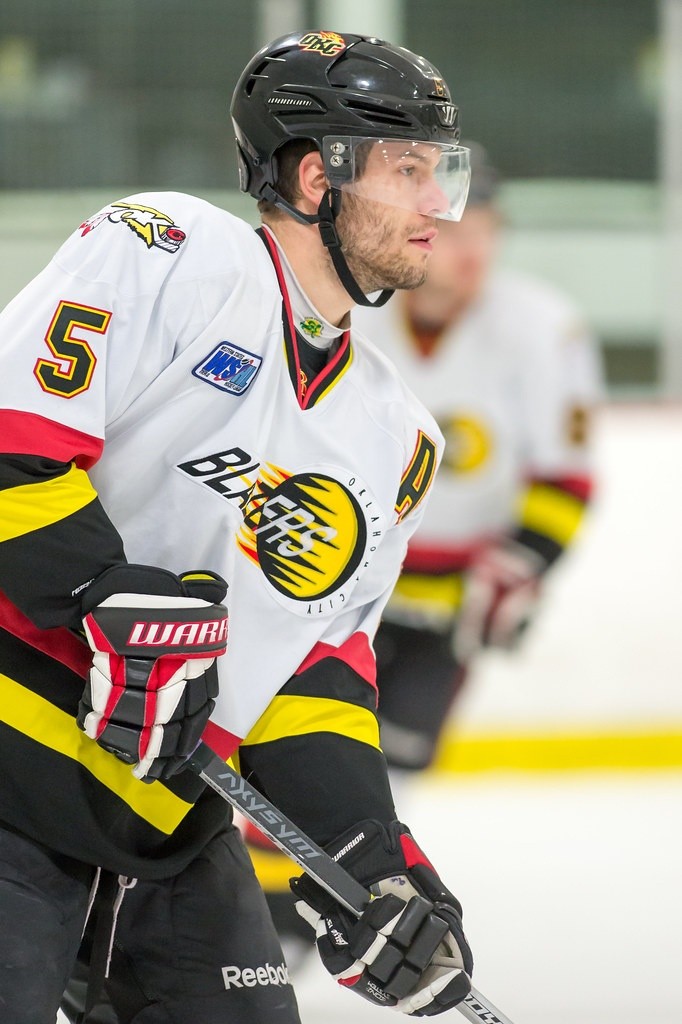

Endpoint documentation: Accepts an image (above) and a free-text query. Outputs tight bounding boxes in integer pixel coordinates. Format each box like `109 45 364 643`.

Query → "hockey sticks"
188 741 510 1024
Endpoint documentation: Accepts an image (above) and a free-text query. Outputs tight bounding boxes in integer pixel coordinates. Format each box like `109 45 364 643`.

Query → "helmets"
227 31 460 203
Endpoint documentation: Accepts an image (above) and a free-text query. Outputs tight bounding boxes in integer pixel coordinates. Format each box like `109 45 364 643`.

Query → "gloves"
451 553 547 667
61 563 229 784
287 819 473 1019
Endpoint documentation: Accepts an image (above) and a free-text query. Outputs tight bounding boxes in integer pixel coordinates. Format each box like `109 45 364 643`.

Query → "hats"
445 141 492 203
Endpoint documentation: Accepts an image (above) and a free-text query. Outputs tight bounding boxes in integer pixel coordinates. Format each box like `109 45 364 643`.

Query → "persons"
0 27 474 1024
232 139 611 983
